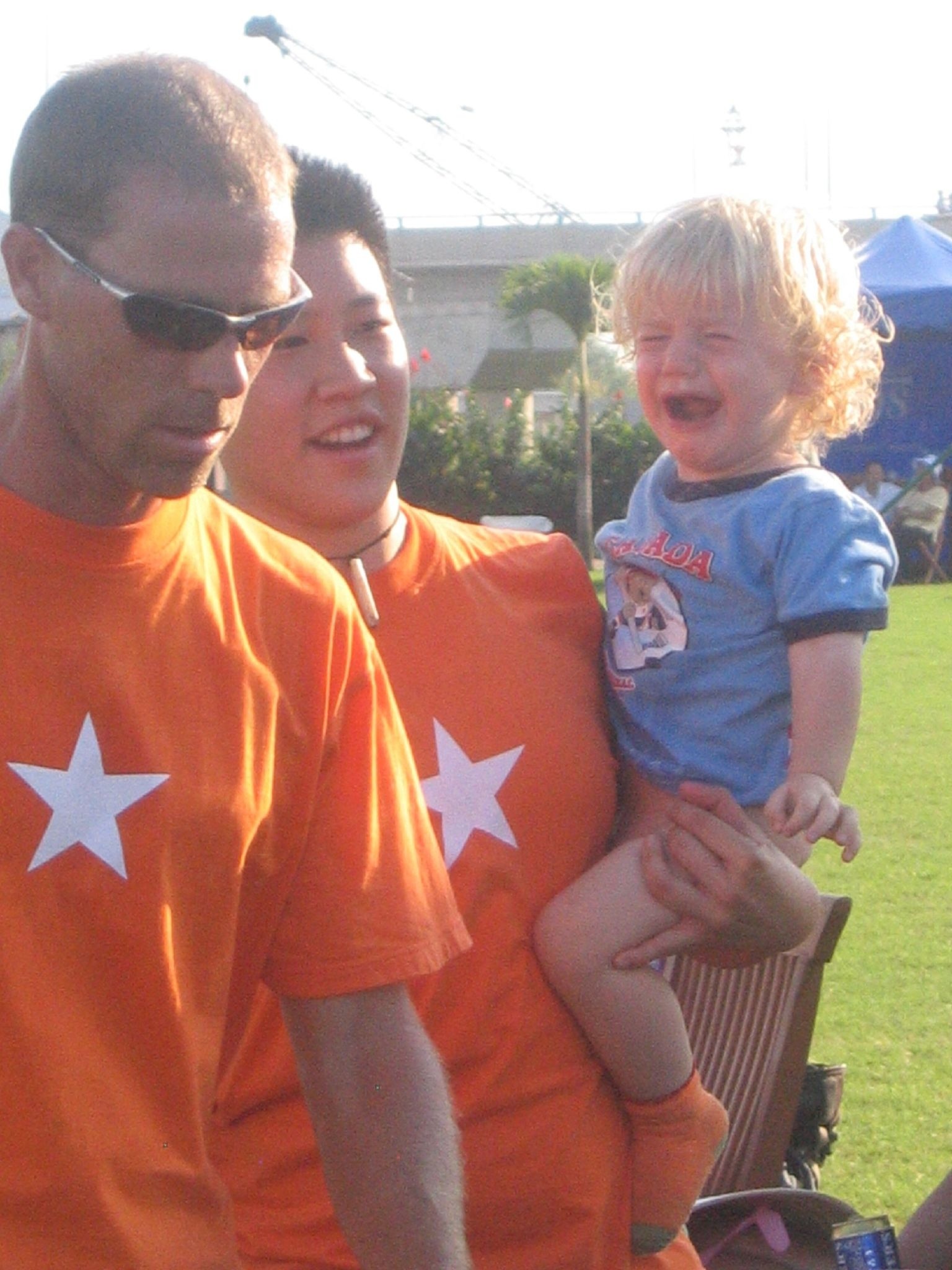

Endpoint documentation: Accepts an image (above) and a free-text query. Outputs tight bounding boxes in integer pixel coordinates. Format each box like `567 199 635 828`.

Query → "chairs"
837 473 952 584
665 894 852 1270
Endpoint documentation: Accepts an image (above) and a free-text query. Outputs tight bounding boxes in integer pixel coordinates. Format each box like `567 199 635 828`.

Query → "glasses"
28 226 317 356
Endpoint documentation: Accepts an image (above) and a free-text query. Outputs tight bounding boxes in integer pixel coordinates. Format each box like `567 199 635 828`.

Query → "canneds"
832 1214 901 1270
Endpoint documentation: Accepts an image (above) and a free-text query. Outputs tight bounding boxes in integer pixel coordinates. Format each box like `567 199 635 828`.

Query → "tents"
833 213 951 490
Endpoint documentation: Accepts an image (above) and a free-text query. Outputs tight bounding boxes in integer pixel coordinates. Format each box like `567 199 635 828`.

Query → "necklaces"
322 507 403 623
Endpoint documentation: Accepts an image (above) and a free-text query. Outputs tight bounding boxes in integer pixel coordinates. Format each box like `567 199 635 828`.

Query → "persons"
837 454 952 579
896 1169 952 1270
531 199 901 1258
207 146 817 1270
0 55 470 1270
